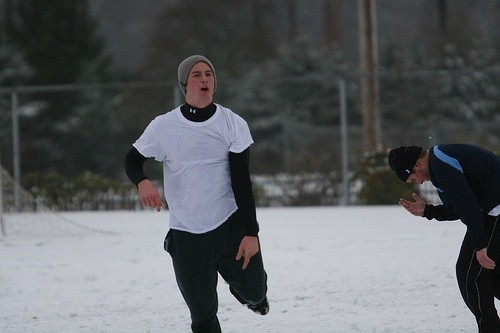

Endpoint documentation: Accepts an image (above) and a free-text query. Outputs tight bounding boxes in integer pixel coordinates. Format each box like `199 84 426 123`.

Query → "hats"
388 145 423 181
178 55 217 97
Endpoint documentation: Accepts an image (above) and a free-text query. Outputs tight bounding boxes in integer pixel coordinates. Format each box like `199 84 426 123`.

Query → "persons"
388 144 500 333
125 53 270 333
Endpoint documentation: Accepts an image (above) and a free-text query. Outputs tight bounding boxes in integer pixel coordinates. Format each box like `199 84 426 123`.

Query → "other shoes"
248 298 270 315
